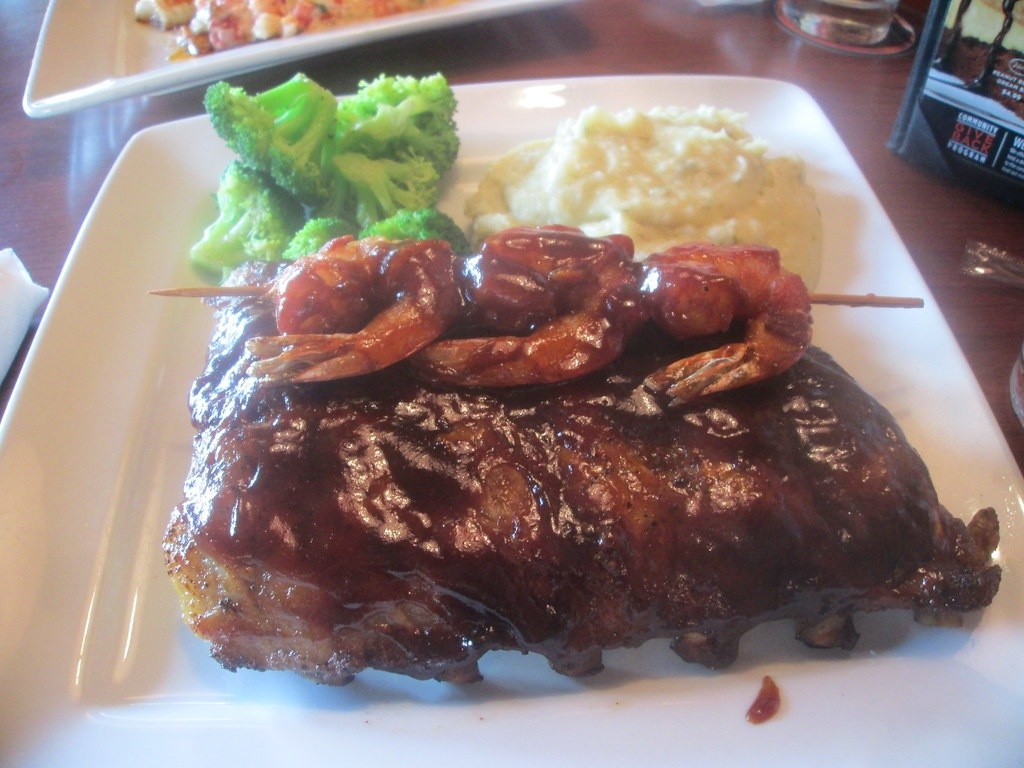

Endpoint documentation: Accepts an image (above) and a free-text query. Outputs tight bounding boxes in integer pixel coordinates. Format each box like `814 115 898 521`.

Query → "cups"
776 0 902 46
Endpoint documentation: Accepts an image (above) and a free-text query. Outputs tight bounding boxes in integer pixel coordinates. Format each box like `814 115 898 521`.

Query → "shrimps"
246 227 815 408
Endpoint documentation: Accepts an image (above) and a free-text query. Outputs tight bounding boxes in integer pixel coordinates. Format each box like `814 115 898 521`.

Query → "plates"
20 0 568 117
0 70 1024 766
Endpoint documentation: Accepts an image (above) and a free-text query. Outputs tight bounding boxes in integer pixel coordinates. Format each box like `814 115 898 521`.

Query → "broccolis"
184 72 475 279
